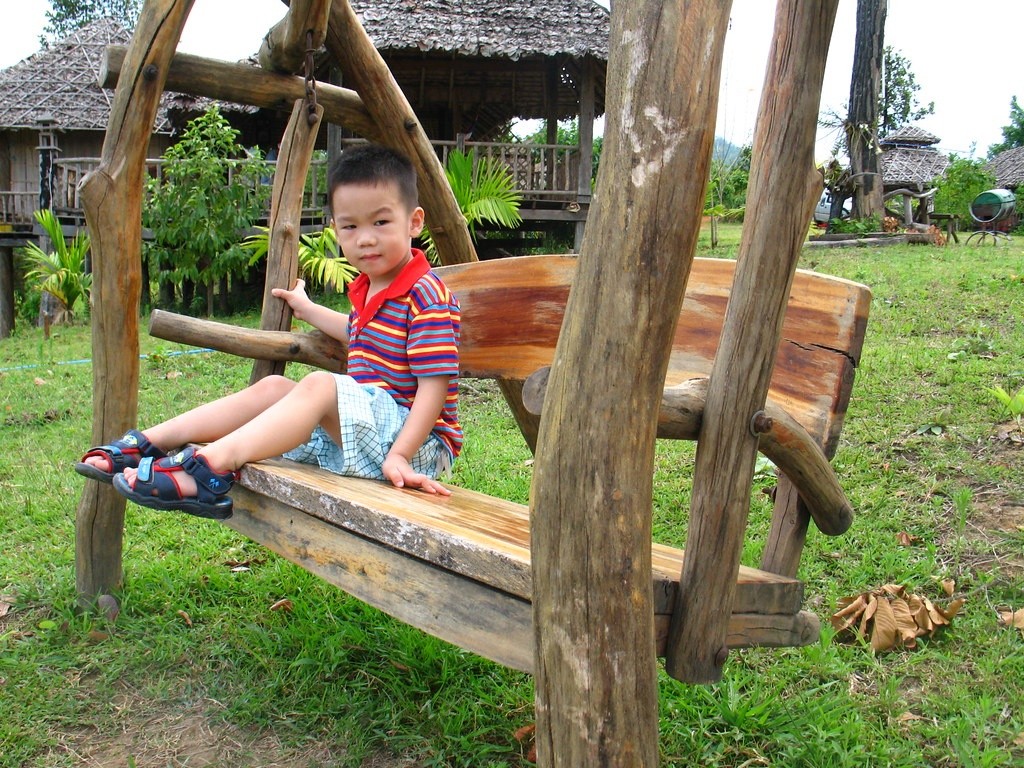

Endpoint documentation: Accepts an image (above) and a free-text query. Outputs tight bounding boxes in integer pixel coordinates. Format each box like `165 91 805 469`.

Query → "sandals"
112 447 240 520
74 428 169 484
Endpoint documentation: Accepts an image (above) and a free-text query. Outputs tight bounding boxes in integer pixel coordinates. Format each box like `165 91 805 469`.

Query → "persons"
75 142 463 517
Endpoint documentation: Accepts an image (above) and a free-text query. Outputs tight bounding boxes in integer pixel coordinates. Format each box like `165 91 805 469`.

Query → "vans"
811 178 854 224
